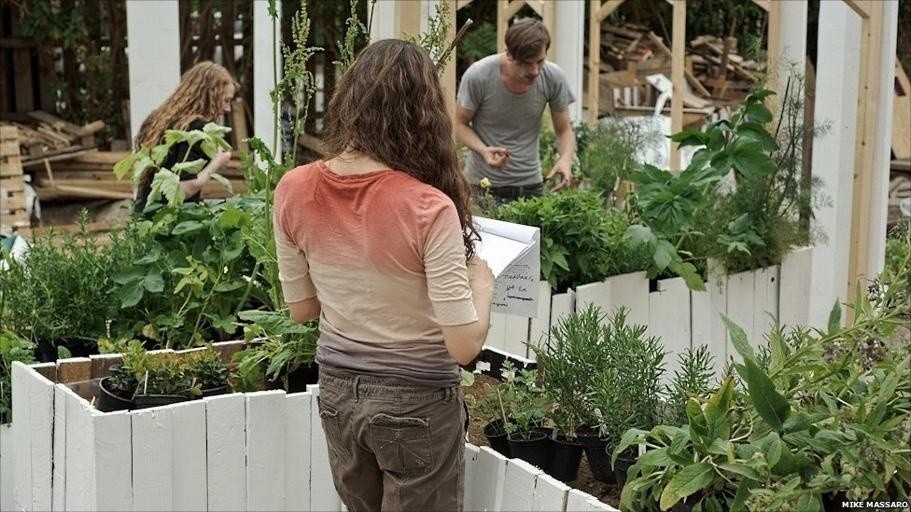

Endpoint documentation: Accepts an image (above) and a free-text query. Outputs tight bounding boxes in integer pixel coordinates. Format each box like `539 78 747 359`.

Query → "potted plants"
463 294 910 508
0 120 320 423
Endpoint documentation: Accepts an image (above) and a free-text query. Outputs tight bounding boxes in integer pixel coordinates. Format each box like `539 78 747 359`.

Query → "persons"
132 60 241 223
452 17 576 205
270 37 496 512
